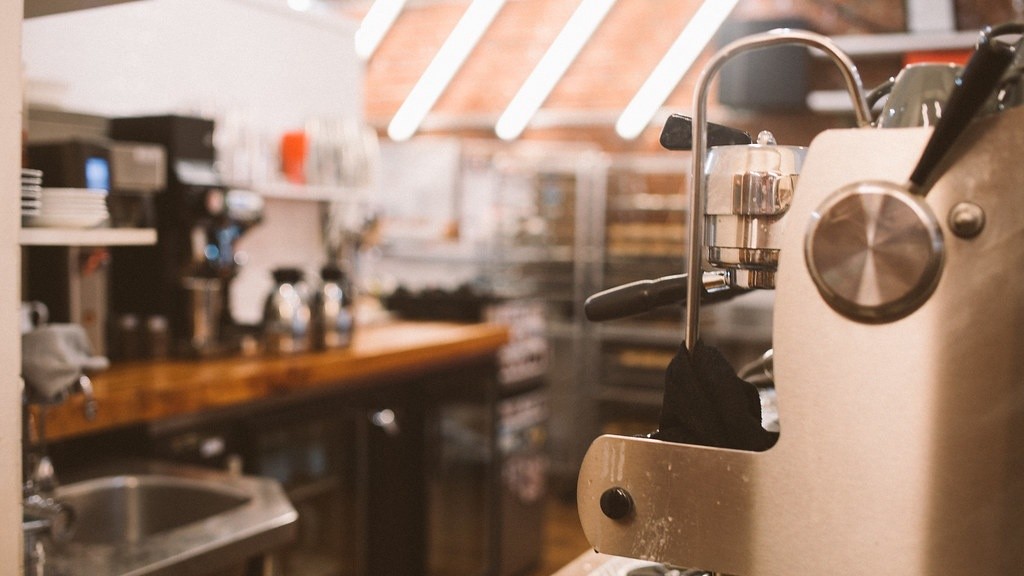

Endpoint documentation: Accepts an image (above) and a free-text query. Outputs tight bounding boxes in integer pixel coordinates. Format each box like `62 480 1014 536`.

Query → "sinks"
35 466 257 560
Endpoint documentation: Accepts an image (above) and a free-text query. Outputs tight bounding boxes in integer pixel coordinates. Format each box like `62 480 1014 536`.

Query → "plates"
20 168 109 228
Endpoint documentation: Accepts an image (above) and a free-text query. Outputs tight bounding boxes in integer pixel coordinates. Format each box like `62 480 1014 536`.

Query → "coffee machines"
107 114 266 358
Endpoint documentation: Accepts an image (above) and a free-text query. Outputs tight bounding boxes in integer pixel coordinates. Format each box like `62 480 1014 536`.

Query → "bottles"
264 266 353 354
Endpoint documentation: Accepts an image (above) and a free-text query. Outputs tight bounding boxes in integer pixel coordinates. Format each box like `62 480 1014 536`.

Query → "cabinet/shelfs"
371 137 693 505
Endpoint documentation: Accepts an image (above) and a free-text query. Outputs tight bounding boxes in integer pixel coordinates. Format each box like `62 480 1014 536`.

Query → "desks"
22 317 514 575
